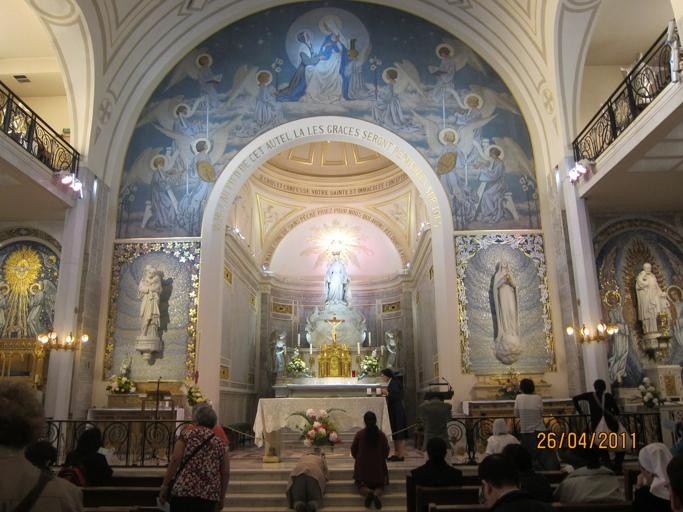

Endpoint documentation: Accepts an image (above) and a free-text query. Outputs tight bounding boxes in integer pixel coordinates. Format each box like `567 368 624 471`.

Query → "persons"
636 262 662 335
479 418 683 512
406 438 462 512
493 257 520 341
667 285 683 365
605 291 628 380
65 429 113 482
27 286 43 336
514 379 547 441
286 455 329 512
324 251 350 304
138 268 163 335
25 440 57 470
381 369 408 461
573 380 625 475
0 286 7 334
159 407 230 512
327 313 342 343
0 379 84 512
350 412 390 508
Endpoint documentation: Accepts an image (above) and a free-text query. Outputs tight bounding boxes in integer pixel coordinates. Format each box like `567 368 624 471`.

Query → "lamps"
49 167 84 200
32 328 90 353
564 319 621 348
566 158 596 184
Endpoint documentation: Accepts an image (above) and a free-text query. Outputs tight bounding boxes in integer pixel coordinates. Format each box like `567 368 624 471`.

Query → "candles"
309 343 312 355
375 387 382 395
380 345 384 356
366 387 372 395
297 333 300 346
282 345 286 357
368 331 372 347
356 340 361 357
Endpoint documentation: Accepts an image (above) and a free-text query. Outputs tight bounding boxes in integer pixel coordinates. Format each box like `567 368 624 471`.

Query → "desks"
249 393 396 464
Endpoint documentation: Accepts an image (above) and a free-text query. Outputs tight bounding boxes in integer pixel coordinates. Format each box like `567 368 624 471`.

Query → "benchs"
73 470 169 511
402 459 652 512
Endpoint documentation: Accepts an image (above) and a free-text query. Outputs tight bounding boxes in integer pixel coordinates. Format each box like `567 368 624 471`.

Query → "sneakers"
373 495 382 510
364 491 372 508
293 501 305 512
387 454 405 461
306 500 318 512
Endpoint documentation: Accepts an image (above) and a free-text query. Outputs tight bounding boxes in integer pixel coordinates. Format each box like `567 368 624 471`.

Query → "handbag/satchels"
594 416 632 452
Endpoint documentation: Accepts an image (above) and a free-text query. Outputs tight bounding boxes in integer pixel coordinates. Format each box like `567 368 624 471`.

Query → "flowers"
633 375 667 411
286 356 308 377
356 353 383 381
453 232 557 375
180 376 208 407
99 358 137 396
99 241 201 382
283 407 346 450
492 366 522 403
